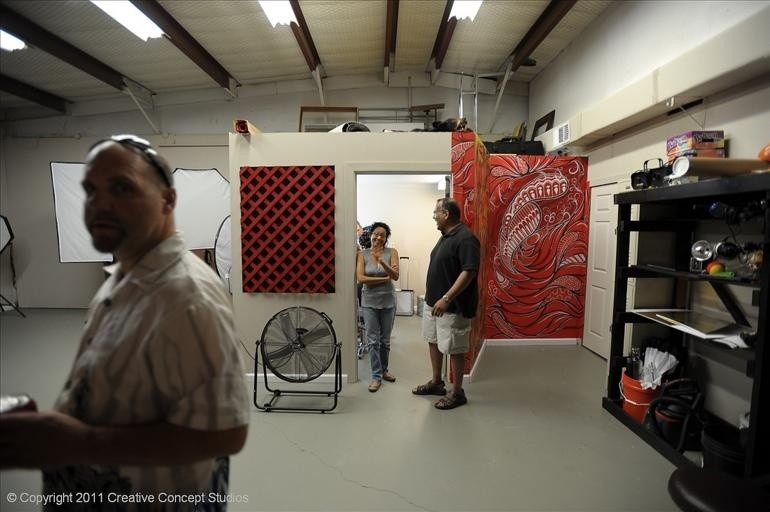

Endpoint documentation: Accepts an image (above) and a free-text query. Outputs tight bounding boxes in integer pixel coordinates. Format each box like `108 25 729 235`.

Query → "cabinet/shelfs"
602 171 770 471
581 178 677 365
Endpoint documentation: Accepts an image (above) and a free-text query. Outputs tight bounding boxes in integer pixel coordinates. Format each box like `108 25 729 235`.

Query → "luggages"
394 257 415 316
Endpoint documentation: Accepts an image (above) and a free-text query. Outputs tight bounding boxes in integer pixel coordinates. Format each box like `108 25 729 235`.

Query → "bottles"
0 394 36 414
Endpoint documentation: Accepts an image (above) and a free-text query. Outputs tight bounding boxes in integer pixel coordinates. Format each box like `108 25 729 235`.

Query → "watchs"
442 295 450 306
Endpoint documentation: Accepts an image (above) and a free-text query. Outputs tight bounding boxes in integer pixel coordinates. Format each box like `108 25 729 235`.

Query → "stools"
668 459 770 512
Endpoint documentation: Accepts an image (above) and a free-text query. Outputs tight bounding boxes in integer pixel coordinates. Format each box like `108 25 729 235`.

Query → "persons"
356 222 400 393
410 198 479 410
358 226 375 249
0 133 252 512
356 221 362 240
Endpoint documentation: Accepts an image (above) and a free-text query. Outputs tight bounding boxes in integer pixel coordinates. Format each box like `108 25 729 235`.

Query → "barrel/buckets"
618 370 657 423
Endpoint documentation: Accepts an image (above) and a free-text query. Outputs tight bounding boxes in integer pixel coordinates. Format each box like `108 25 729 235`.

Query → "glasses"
371 232 388 239
433 209 449 215
89 133 174 187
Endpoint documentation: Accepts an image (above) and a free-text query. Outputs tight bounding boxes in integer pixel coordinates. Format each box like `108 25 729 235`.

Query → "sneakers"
382 374 396 382
369 377 381 391
435 388 468 409
411 380 447 395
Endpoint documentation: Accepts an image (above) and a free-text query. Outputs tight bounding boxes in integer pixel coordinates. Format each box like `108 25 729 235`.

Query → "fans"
250 304 344 416
